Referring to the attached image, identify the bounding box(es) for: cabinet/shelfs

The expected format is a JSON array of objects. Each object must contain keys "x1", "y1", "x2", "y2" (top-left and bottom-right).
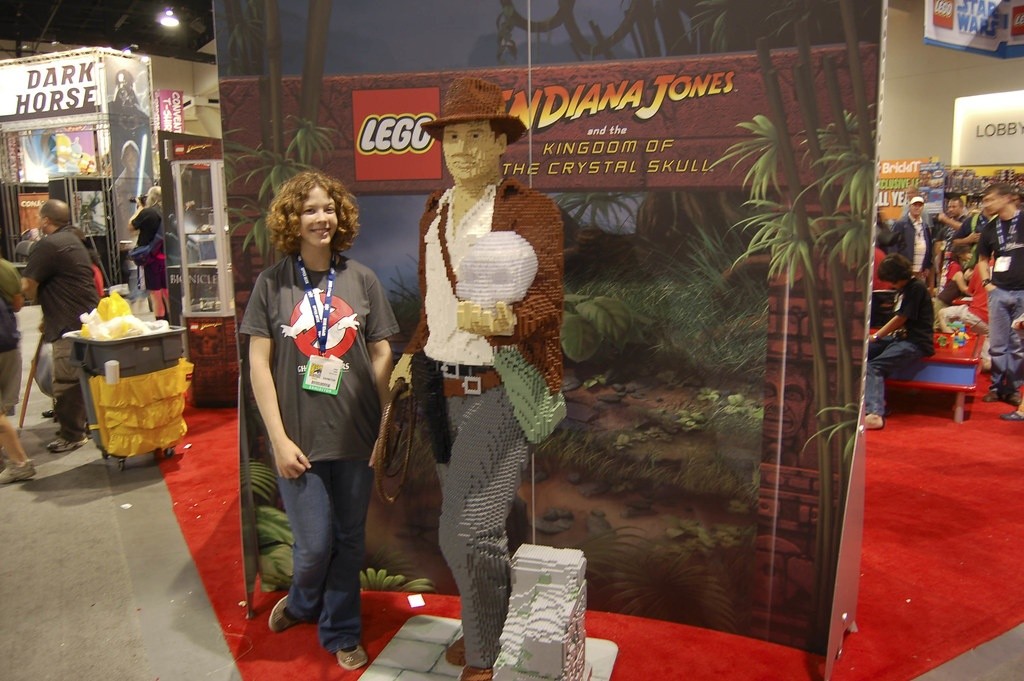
[{"x1": 172, "y1": 160, "x2": 236, "y2": 318}]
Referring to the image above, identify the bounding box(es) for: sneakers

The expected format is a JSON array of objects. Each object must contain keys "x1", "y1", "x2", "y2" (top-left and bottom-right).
[
  {"x1": 46, "y1": 435, "x2": 88, "y2": 452},
  {"x1": 0, "y1": 458, "x2": 37, "y2": 484},
  {"x1": 56, "y1": 421, "x2": 93, "y2": 439}
]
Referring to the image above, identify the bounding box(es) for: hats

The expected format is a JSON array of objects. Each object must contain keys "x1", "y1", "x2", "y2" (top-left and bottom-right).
[{"x1": 910, "y1": 196, "x2": 925, "y2": 205}]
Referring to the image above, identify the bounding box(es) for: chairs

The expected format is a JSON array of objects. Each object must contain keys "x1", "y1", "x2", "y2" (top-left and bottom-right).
[{"x1": 871, "y1": 289, "x2": 897, "y2": 327}]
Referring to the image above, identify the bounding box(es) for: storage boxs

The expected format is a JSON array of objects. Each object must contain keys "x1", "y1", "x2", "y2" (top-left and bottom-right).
[{"x1": 61, "y1": 326, "x2": 187, "y2": 378}]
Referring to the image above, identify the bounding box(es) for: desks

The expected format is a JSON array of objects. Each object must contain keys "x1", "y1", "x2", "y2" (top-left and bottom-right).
[{"x1": 870, "y1": 327, "x2": 985, "y2": 424}]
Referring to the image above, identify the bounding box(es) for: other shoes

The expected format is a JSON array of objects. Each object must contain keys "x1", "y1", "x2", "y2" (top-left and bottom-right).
[
  {"x1": 1005, "y1": 393, "x2": 1022, "y2": 405},
  {"x1": 268, "y1": 595, "x2": 301, "y2": 633},
  {"x1": 42, "y1": 409, "x2": 55, "y2": 417},
  {"x1": 337, "y1": 644, "x2": 368, "y2": 670},
  {"x1": 1000, "y1": 412, "x2": 1024, "y2": 420},
  {"x1": 983, "y1": 390, "x2": 1001, "y2": 402},
  {"x1": 864, "y1": 412, "x2": 883, "y2": 430}
]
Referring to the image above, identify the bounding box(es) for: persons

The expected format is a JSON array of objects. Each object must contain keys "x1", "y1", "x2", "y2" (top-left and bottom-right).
[
  {"x1": 114, "y1": 140, "x2": 151, "y2": 245},
  {"x1": 0, "y1": 199, "x2": 102, "y2": 483},
  {"x1": 388, "y1": 74, "x2": 568, "y2": 681},
  {"x1": 863, "y1": 182, "x2": 1024, "y2": 430},
  {"x1": 245, "y1": 173, "x2": 394, "y2": 671},
  {"x1": 127, "y1": 186, "x2": 169, "y2": 322},
  {"x1": 107, "y1": 70, "x2": 153, "y2": 174}
]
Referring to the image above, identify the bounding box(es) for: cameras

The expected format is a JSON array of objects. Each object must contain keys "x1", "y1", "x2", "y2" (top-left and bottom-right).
[{"x1": 130, "y1": 194, "x2": 148, "y2": 206}]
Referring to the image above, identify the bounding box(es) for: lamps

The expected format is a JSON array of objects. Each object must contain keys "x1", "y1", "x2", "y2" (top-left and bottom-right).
[
  {"x1": 122, "y1": 44, "x2": 139, "y2": 53},
  {"x1": 51, "y1": 41, "x2": 71, "y2": 51}
]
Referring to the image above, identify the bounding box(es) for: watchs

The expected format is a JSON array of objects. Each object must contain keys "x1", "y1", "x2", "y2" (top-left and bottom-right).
[
  {"x1": 872, "y1": 332, "x2": 881, "y2": 341},
  {"x1": 980, "y1": 279, "x2": 991, "y2": 287}
]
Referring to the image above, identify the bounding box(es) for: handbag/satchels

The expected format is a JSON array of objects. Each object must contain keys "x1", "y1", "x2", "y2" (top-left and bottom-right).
[
  {"x1": 937, "y1": 280, "x2": 961, "y2": 307},
  {"x1": 958, "y1": 214, "x2": 980, "y2": 269},
  {"x1": 127, "y1": 244, "x2": 154, "y2": 267}
]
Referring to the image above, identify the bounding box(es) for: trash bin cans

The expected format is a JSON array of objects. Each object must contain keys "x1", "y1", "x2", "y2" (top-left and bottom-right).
[{"x1": 62, "y1": 327, "x2": 188, "y2": 459}]
